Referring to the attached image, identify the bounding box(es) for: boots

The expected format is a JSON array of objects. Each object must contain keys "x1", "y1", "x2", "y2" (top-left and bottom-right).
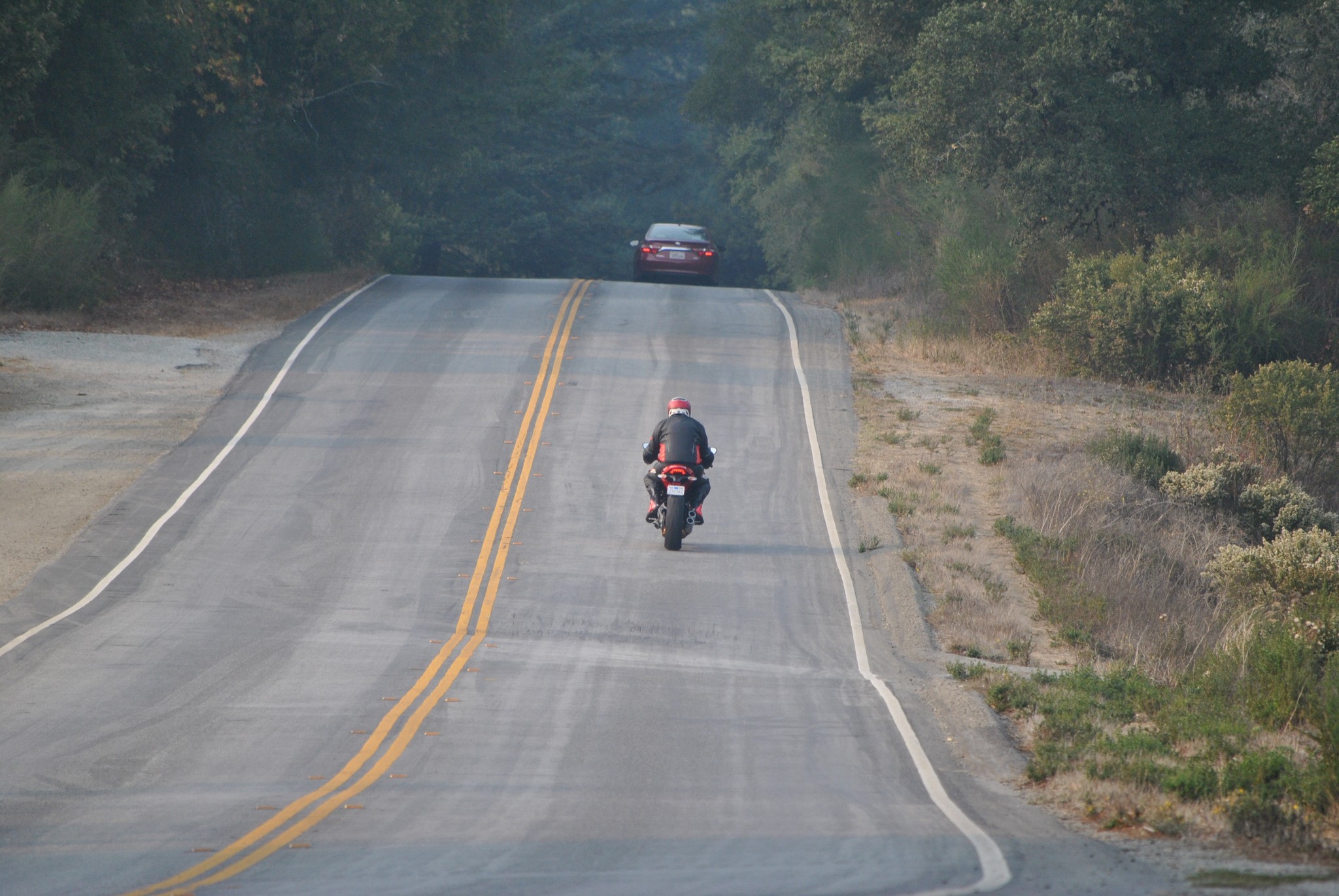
[
  {"x1": 646, "y1": 500, "x2": 657, "y2": 523},
  {"x1": 694, "y1": 504, "x2": 704, "y2": 525}
]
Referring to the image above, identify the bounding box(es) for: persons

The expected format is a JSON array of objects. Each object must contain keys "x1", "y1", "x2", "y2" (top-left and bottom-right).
[{"x1": 642, "y1": 396, "x2": 714, "y2": 524}]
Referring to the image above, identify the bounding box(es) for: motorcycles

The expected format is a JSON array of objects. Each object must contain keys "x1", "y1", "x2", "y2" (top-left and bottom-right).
[{"x1": 642, "y1": 441, "x2": 717, "y2": 551}]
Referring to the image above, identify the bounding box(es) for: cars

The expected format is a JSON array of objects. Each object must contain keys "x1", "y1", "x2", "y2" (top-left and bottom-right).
[{"x1": 630, "y1": 222, "x2": 726, "y2": 288}]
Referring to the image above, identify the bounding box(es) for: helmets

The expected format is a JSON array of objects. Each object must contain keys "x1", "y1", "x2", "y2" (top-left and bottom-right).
[{"x1": 668, "y1": 397, "x2": 692, "y2": 417}]
